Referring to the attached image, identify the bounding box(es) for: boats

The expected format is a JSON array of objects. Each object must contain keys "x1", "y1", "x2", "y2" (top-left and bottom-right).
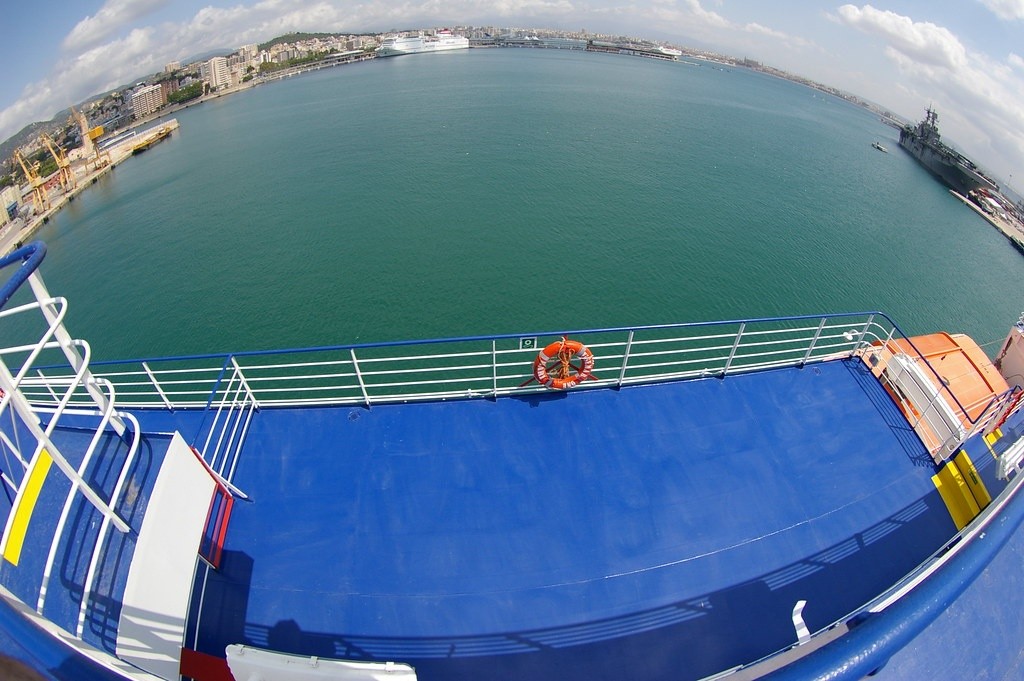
[
  {"x1": 133, "y1": 126, "x2": 171, "y2": 151},
  {"x1": 871, "y1": 141, "x2": 887, "y2": 153}
]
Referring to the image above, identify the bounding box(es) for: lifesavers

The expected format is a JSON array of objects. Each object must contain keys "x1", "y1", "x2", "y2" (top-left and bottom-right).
[{"x1": 533, "y1": 339, "x2": 594, "y2": 389}]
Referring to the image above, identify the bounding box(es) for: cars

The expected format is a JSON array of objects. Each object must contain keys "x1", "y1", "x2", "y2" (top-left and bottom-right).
[{"x1": 968, "y1": 185, "x2": 1024, "y2": 227}]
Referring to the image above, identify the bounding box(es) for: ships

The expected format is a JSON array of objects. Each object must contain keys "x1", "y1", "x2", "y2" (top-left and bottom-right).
[
  {"x1": 901, "y1": 104, "x2": 1000, "y2": 200},
  {"x1": 0, "y1": 236, "x2": 1024, "y2": 681},
  {"x1": 375, "y1": 31, "x2": 470, "y2": 57}
]
